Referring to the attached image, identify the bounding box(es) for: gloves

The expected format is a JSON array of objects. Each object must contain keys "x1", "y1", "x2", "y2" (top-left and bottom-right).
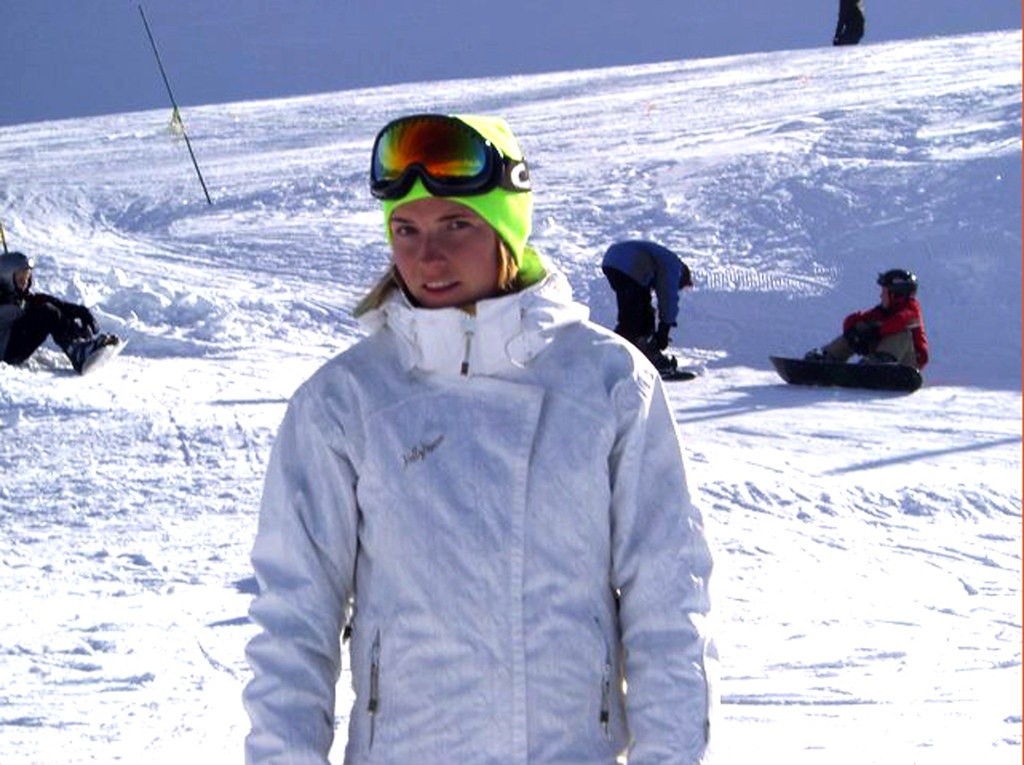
[
  {"x1": 855, "y1": 321, "x2": 881, "y2": 342},
  {"x1": 77, "y1": 305, "x2": 100, "y2": 334},
  {"x1": 655, "y1": 321, "x2": 671, "y2": 350},
  {"x1": 842, "y1": 325, "x2": 871, "y2": 356}
]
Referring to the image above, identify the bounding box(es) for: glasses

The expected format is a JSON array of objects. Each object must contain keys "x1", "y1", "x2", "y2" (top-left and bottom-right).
[{"x1": 368, "y1": 112, "x2": 533, "y2": 201}]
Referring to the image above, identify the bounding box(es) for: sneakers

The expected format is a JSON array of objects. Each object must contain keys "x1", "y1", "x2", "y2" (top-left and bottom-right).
[
  {"x1": 646, "y1": 339, "x2": 671, "y2": 372},
  {"x1": 66, "y1": 334, "x2": 107, "y2": 376},
  {"x1": 95, "y1": 329, "x2": 119, "y2": 374}
]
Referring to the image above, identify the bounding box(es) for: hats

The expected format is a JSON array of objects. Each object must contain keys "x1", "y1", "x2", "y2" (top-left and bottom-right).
[{"x1": 383, "y1": 116, "x2": 535, "y2": 269}]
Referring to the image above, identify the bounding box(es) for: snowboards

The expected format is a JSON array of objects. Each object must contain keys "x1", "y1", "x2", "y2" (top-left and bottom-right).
[
  {"x1": 659, "y1": 369, "x2": 695, "y2": 382},
  {"x1": 767, "y1": 356, "x2": 922, "y2": 394},
  {"x1": 80, "y1": 337, "x2": 132, "y2": 375}
]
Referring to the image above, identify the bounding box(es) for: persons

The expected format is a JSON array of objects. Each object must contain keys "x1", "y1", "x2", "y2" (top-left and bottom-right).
[
  {"x1": 600, "y1": 239, "x2": 693, "y2": 373},
  {"x1": 242, "y1": 110, "x2": 720, "y2": 765},
  {"x1": 832, "y1": 0, "x2": 866, "y2": 46},
  {"x1": 0, "y1": 251, "x2": 125, "y2": 377},
  {"x1": 803, "y1": 268, "x2": 930, "y2": 369}
]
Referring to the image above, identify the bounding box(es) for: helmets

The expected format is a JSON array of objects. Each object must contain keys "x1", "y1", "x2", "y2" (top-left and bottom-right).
[
  {"x1": 876, "y1": 268, "x2": 918, "y2": 298},
  {"x1": 0, "y1": 251, "x2": 34, "y2": 295}
]
